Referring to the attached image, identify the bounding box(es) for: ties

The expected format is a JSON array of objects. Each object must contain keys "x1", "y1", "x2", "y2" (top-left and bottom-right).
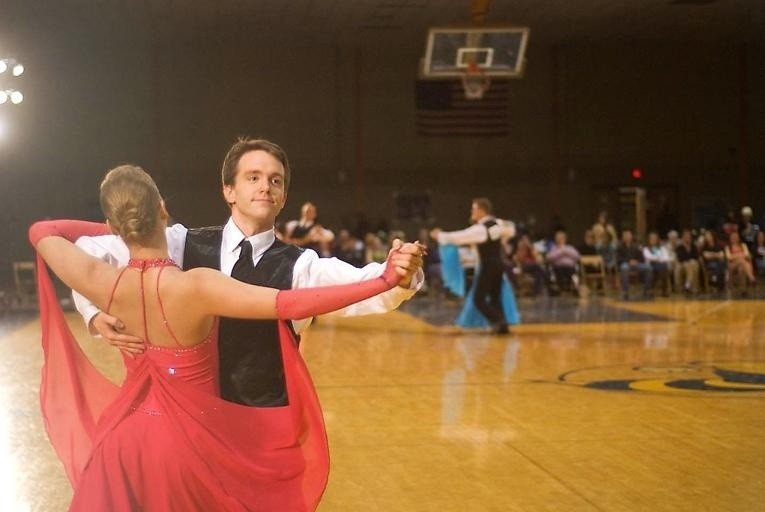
[{"x1": 231, "y1": 241, "x2": 254, "y2": 283}]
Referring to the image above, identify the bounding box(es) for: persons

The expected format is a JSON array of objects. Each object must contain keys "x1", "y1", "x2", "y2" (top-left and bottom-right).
[
  {"x1": 71, "y1": 136, "x2": 426, "y2": 408},
  {"x1": 284, "y1": 199, "x2": 759, "y2": 332},
  {"x1": 31, "y1": 165, "x2": 423, "y2": 512}
]
